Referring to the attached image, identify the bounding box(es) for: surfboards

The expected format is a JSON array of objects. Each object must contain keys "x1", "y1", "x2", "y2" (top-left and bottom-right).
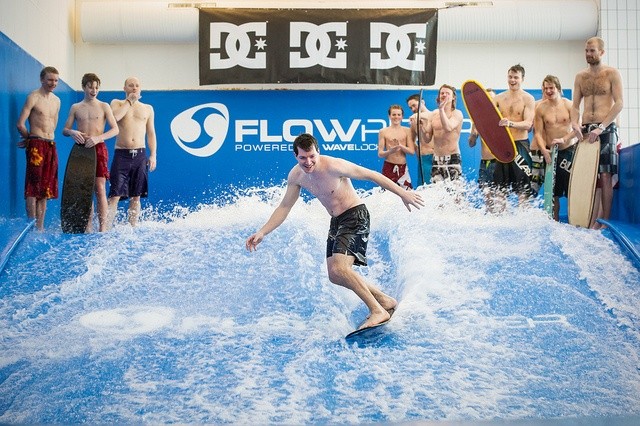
[
  {"x1": 544, "y1": 144, "x2": 559, "y2": 221},
  {"x1": 344, "y1": 306, "x2": 396, "y2": 339},
  {"x1": 460, "y1": 79, "x2": 518, "y2": 164},
  {"x1": 567, "y1": 132, "x2": 601, "y2": 230},
  {"x1": 416, "y1": 88, "x2": 425, "y2": 186},
  {"x1": 60, "y1": 142, "x2": 97, "y2": 235}
]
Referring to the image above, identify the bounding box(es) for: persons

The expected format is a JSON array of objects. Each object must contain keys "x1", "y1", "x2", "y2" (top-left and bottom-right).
[
  {"x1": 572, "y1": 35, "x2": 623, "y2": 231},
  {"x1": 246, "y1": 132, "x2": 426, "y2": 331},
  {"x1": 16, "y1": 65, "x2": 61, "y2": 231},
  {"x1": 108, "y1": 76, "x2": 157, "y2": 230},
  {"x1": 488, "y1": 64, "x2": 535, "y2": 211},
  {"x1": 418, "y1": 83, "x2": 463, "y2": 179},
  {"x1": 528, "y1": 82, "x2": 552, "y2": 201},
  {"x1": 468, "y1": 88, "x2": 503, "y2": 184},
  {"x1": 377, "y1": 103, "x2": 415, "y2": 192},
  {"x1": 405, "y1": 94, "x2": 435, "y2": 182},
  {"x1": 62, "y1": 71, "x2": 121, "y2": 231},
  {"x1": 535, "y1": 74, "x2": 582, "y2": 223}
]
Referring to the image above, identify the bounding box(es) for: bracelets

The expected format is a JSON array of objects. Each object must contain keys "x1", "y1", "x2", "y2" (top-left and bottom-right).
[{"x1": 509, "y1": 121, "x2": 514, "y2": 127}]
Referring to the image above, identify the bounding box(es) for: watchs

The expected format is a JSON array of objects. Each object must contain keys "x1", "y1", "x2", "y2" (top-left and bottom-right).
[{"x1": 598, "y1": 124, "x2": 606, "y2": 131}]
